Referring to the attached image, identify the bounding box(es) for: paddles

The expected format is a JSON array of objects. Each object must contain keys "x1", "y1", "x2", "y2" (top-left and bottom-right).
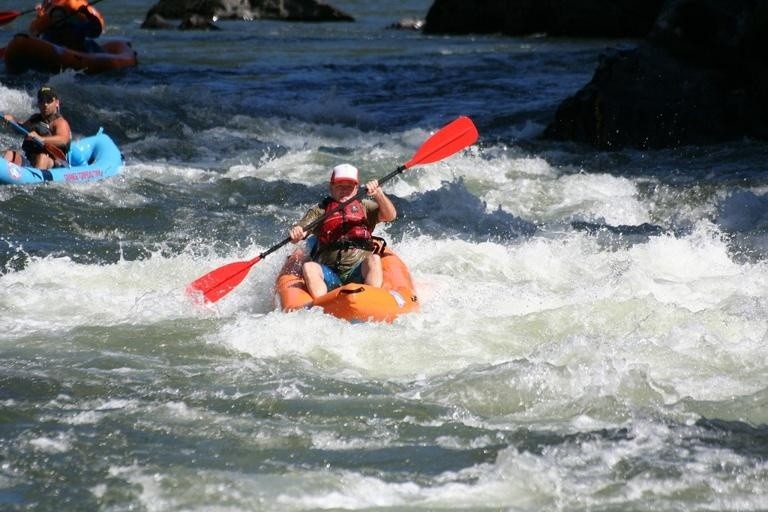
[
  {"x1": 0, "y1": 113, "x2": 66, "y2": 161},
  {"x1": 1, "y1": 9, "x2": 35, "y2": 26},
  {"x1": 188, "y1": 115, "x2": 479, "y2": 305}
]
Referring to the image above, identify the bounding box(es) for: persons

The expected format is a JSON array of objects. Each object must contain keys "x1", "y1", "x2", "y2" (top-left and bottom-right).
[
  {"x1": 288, "y1": 164, "x2": 397, "y2": 301},
  {"x1": 3, "y1": 87, "x2": 72, "y2": 170},
  {"x1": 30, "y1": 0, "x2": 103, "y2": 54}
]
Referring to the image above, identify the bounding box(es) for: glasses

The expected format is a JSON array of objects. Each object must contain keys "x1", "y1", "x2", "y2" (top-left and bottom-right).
[{"x1": 41, "y1": 99, "x2": 54, "y2": 104}]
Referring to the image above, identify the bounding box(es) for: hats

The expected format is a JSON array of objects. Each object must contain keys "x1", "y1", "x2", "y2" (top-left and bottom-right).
[
  {"x1": 38, "y1": 87, "x2": 57, "y2": 102},
  {"x1": 331, "y1": 164, "x2": 361, "y2": 185}
]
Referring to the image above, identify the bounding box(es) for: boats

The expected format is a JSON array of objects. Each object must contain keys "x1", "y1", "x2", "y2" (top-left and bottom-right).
[
  {"x1": 274, "y1": 234, "x2": 419, "y2": 327},
  {"x1": 1, "y1": 33, "x2": 138, "y2": 78},
  {"x1": 0, "y1": 129, "x2": 122, "y2": 186}
]
